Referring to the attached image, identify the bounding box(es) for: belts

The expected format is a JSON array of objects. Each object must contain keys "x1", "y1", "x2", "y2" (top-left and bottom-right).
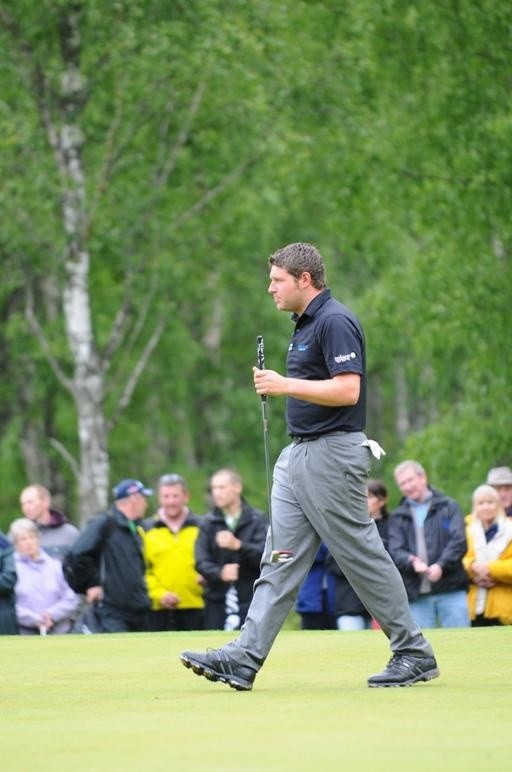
[{"x1": 292, "y1": 430, "x2": 336, "y2": 444}]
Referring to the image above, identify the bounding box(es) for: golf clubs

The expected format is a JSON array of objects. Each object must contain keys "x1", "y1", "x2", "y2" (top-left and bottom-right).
[{"x1": 254, "y1": 334, "x2": 295, "y2": 565}]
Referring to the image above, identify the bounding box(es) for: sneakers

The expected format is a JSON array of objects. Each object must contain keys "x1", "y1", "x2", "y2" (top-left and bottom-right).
[
  {"x1": 179, "y1": 647, "x2": 256, "y2": 691},
  {"x1": 366, "y1": 654, "x2": 440, "y2": 687}
]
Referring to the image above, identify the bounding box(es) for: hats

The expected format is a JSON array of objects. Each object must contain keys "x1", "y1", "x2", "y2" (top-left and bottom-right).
[
  {"x1": 486, "y1": 466, "x2": 512, "y2": 486},
  {"x1": 112, "y1": 478, "x2": 155, "y2": 500}
]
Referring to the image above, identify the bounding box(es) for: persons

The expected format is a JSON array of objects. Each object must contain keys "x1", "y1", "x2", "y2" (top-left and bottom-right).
[
  {"x1": 294, "y1": 460, "x2": 512, "y2": 631},
  {"x1": 0, "y1": 469, "x2": 268, "y2": 635},
  {"x1": 179, "y1": 242, "x2": 442, "y2": 693}
]
